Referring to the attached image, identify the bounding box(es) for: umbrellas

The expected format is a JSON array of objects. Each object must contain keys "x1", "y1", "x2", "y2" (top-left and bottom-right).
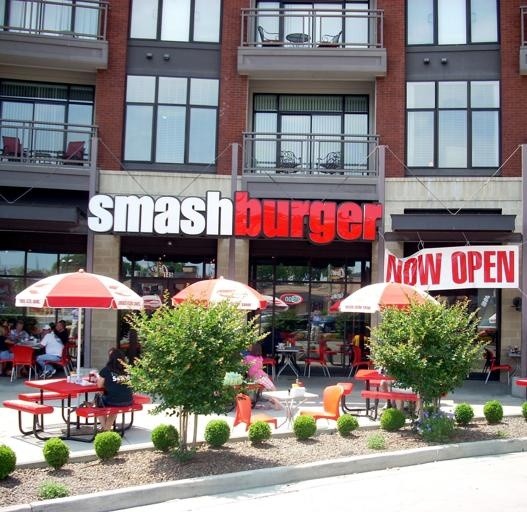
[
  {"x1": 167, "y1": 275, "x2": 268, "y2": 314},
  {"x1": 14, "y1": 268, "x2": 148, "y2": 436},
  {"x1": 262, "y1": 293, "x2": 291, "y2": 313},
  {"x1": 141, "y1": 292, "x2": 162, "y2": 308},
  {"x1": 327, "y1": 281, "x2": 446, "y2": 408}
]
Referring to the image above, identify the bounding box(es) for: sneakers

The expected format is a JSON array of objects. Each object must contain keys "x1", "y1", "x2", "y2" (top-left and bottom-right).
[{"x1": 46, "y1": 368, "x2": 56, "y2": 378}]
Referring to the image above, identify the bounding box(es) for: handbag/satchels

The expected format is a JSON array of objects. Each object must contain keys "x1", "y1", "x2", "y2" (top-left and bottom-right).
[{"x1": 94, "y1": 393, "x2": 104, "y2": 408}]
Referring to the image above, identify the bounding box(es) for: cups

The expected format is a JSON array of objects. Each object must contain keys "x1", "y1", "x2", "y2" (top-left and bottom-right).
[{"x1": 67, "y1": 376, "x2": 71, "y2": 383}]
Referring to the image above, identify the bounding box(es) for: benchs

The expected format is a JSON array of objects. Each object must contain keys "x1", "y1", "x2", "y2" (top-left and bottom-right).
[
  {"x1": 337, "y1": 382, "x2": 423, "y2": 419},
  {"x1": 2, "y1": 391, "x2": 152, "y2": 445}
]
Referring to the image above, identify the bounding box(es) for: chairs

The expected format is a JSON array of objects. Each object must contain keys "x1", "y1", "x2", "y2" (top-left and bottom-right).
[
  {"x1": 233, "y1": 392, "x2": 278, "y2": 433},
  {"x1": 0, "y1": 340, "x2": 80, "y2": 382},
  {"x1": 55, "y1": 140, "x2": 84, "y2": 166},
  {"x1": 481, "y1": 346, "x2": 512, "y2": 385},
  {"x1": 300, "y1": 382, "x2": 345, "y2": 425},
  {"x1": 313, "y1": 30, "x2": 344, "y2": 48},
  {"x1": 258, "y1": 339, "x2": 373, "y2": 382},
  {"x1": 0, "y1": 134, "x2": 28, "y2": 161},
  {"x1": 314, "y1": 152, "x2": 342, "y2": 176},
  {"x1": 276, "y1": 150, "x2": 302, "y2": 173},
  {"x1": 256, "y1": 26, "x2": 284, "y2": 49}
]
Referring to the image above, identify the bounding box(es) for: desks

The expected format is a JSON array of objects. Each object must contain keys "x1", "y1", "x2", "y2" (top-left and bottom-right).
[
  {"x1": 286, "y1": 33, "x2": 310, "y2": 48},
  {"x1": 24, "y1": 376, "x2": 107, "y2": 437},
  {"x1": 260, "y1": 387, "x2": 319, "y2": 430},
  {"x1": 352, "y1": 368, "x2": 402, "y2": 419}
]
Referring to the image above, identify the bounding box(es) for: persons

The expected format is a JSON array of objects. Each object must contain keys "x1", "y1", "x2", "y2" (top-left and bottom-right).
[
  {"x1": 82, "y1": 347, "x2": 114, "y2": 409},
  {"x1": 377, "y1": 361, "x2": 420, "y2": 412},
  {"x1": 241, "y1": 343, "x2": 283, "y2": 410},
  {"x1": 91, "y1": 347, "x2": 132, "y2": 432},
  {"x1": 0, "y1": 319, "x2": 68, "y2": 379},
  {"x1": 261, "y1": 328, "x2": 371, "y2": 373},
  {"x1": 114, "y1": 325, "x2": 144, "y2": 362}
]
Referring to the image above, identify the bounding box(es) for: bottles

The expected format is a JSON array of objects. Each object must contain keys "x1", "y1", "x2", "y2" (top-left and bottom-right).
[
  {"x1": 89, "y1": 372, "x2": 95, "y2": 384},
  {"x1": 287, "y1": 381, "x2": 305, "y2": 397}
]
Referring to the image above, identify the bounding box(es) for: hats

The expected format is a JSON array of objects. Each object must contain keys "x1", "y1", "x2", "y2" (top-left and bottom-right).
[{"x1": 41, "y1": 324, "x2": 52, "y2": 330}]
